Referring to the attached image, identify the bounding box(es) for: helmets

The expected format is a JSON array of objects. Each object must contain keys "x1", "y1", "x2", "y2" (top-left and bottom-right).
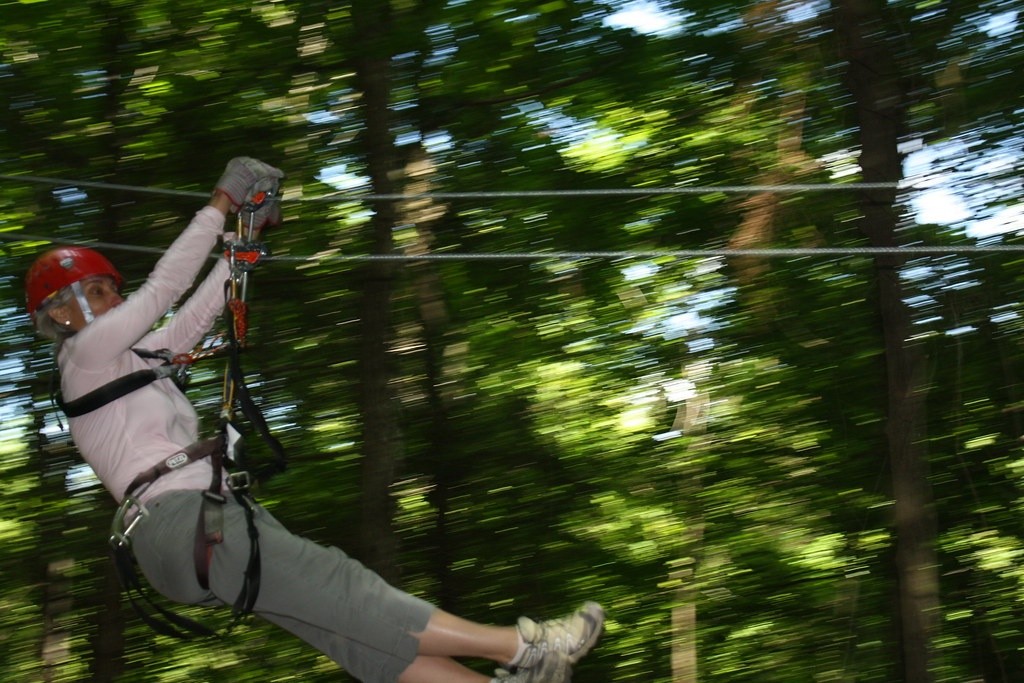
[{"x1": 25, "y1": 246, "x2": 122, "y2": 323}]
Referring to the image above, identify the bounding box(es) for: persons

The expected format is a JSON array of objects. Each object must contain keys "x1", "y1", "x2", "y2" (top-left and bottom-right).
[{"x1": 25, "y1": 156, "x2": 604, "y2": 683}]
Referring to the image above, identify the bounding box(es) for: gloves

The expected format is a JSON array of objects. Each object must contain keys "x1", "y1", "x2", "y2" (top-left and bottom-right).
[
  {"x1": 214, "y1": 156, "x2": 283, "y2": 213},
  {"x1": 240, "y1": 175, "x2": 280, "y2": 229}
]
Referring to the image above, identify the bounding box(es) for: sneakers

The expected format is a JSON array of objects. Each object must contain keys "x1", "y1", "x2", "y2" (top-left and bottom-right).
[
  {"x1": 494, "y1": 652, "x2": 572, "y2": 683},
  {"x1": 511, "y1": 602, "x2": 603, "y2": 668}
]
